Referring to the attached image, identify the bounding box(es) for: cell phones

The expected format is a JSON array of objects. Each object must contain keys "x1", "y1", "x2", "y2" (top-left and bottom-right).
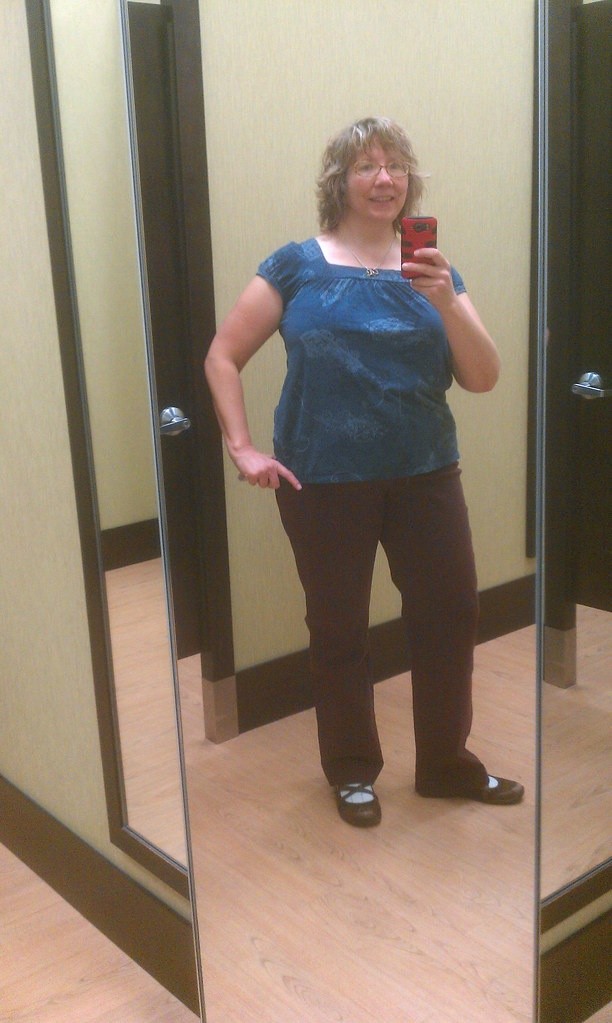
[{"x1": 400, "y1": 216, "x2": 439, "y2": 280}]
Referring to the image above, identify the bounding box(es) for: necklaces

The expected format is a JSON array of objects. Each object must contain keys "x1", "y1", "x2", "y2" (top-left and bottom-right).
[{"x1": 335, "y1": 230, "x2": 398, "y2": 278}]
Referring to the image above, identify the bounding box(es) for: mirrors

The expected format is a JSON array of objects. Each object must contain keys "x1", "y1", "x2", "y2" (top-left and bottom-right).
[
  {"x1": 534, "y1": 0, "x2": 612, "y2": 939},
  {"x1": 118, "y1": 0, "x2": 545, "y2": 1023},
  {"x1": 27, "y1": 1, "x2": 196, "y2": 897}
]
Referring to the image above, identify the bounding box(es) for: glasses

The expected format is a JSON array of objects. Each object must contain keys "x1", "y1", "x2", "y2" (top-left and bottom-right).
[{"x1": 348, "y1": 157, "x2": 412, "y2": 179}]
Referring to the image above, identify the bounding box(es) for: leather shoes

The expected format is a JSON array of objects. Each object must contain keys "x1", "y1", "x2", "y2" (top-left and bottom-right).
[
  {"x1": 334, "y1": 781, "x2": 382, "y2": 829},
  {"x1": 463, "y1": 773, "x2": 525, "y2": 805}
]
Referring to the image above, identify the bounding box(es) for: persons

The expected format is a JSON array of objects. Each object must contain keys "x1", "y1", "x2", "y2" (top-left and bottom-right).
[{"x1": 202, "y1": 116, "x2": 528, "y2": 827}]
